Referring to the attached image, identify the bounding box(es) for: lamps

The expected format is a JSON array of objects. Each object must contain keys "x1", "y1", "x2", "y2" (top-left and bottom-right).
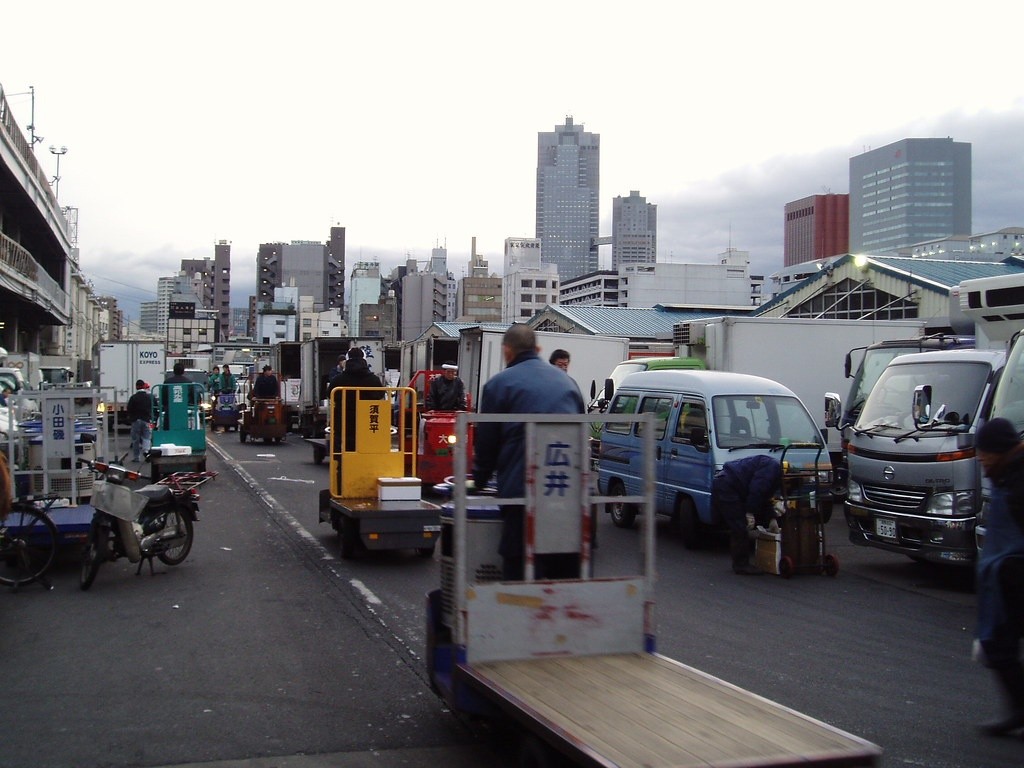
[{"x1": 816, "y1": 261, "x2": 833, "y2": 277}]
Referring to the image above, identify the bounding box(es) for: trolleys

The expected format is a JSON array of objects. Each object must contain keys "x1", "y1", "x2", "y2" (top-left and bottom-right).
[{"x1": 774, "y1": 443, "x2": 836, "y2": 576}]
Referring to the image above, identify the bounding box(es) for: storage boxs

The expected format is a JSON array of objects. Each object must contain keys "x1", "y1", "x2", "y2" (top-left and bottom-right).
[
  {"x1": 151, "y1": 446, "x2": 192, "y2": 455},
  {"x1": 378, "y1": 477, "x2": 422, "y2": 501}
]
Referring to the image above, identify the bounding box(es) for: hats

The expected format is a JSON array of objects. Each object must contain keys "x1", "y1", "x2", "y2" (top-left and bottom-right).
[
  {"x1": 145, "y1": 383, "x2": 150, "y2": 388},
  {"x1": 136, "y1": 379, "x2": 145, "y2": 388}
]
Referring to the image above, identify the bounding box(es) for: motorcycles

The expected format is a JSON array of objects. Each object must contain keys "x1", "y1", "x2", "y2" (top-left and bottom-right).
[{"x1": 78, "y1": 449, "x2": 219, "y2": 591}]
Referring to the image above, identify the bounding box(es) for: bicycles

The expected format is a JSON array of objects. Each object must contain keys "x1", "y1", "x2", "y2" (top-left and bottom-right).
[{"x1": 0, "y1": 493, "x2": 65, "y2": 591}]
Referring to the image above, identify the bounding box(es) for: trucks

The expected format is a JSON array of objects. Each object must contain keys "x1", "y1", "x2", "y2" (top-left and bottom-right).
[
  {"x1": 455, "y1": 272, "x2": 1024, "y2": 590},
  {"x1": 38, "y1": 336, "x2": 402, "y2": 442}
]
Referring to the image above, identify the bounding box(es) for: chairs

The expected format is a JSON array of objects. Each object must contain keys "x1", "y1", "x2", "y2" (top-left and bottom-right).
[
  {"x1": 683, "y1": 411, "x2": 707, "y2": 433},
  {"x1": 730, "y1": 416, "x2": 752, "y2": 438}
]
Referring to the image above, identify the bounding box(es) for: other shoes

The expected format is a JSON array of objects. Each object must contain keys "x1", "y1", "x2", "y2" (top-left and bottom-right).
[
  {"x1": 143, "y1": 452, "x2": 150, "y2": 463},
  {"x1": 738, "y1": 565, "x2": 763, "y2": 576},
  {"x1": 131, "y1": 459, "x2": 139, "y2": 462},
  {"x1": 978, "y1": 717, "x2": 1024, "y2": 741}
]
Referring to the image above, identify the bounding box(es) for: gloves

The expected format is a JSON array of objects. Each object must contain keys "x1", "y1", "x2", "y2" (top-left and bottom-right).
[
  {"x1": 769, "y1": 515, "x2": 781, "y2": 535},
  {"x1": 746, "y1": 512, "x2": 754, "y2": 530}
]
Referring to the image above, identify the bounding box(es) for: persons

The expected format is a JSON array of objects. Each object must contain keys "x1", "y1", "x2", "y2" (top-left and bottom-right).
[
  {"x1": 972, "y1": 417, "x2": 1024, "y2": 731},
  {"x1": 328, "y1": 355, "x2": 347, "y2": 382},
  {"x1": 709, "y1": 455, "x2": 801, "y2": 575},
  {"x1": 425, "y1": 361, "x2": 468, "y2": 412},
  {"x1": 252, "y1": 365, "x2": 281, "y2": 402},
  {"x1": 470, "y1": 323, "x2": 599, "y2": 581},
  {"x1": 549, "y1": 349, "x2": 570, "y2": 373},
  {"x1": 162, "y1": 362, "x2": 202, "y2": 430},
  {"x1": 219, "y1": 364, "x2": 237, "y2": 394},
  {"x1": 2, "y1": 387, "x2": 13, "y2": 398},
  {"x1": 126, "y1": 379, "x2": 159, "y2": 461},
  {"x1": 207, "y1": 366, "x2": 224, "y2": 396},
  {"x1": 327, "y1": 348, "x2": 385, "y2": 495}
]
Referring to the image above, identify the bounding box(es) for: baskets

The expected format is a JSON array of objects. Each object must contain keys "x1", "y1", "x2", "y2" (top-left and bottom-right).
[{"x1": 90, "y1": 481, "x2": 149, "y2": 521}]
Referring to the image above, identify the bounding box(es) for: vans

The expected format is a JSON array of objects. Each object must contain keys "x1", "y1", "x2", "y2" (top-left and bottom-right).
[{"x1": 598, "y1": 369, "x2": 835, "y2": 548}]
